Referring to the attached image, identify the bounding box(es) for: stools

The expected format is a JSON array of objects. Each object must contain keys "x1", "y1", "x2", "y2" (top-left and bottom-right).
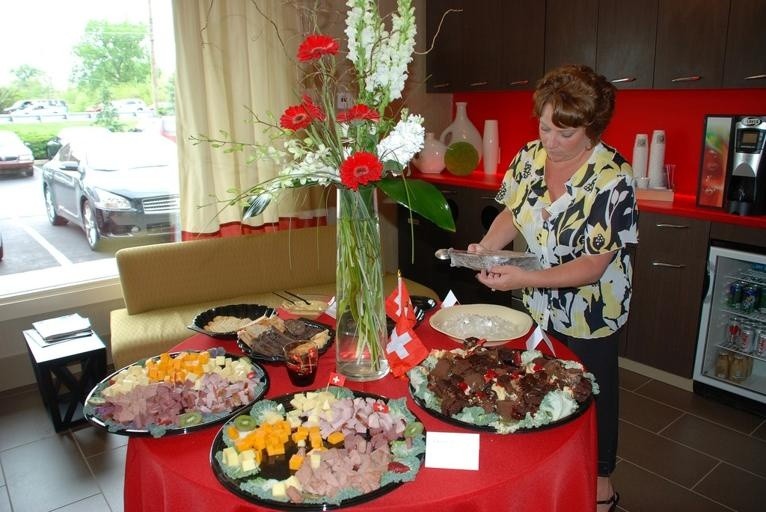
[{"x1": 21, "y1": 329, "x2": 107, "y2": 434}]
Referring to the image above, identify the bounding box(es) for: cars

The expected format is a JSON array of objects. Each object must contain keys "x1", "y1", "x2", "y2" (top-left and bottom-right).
[
  {"x1": 115, "y1": 99, "x2": 147, "y2": 111},
  {"x1": 42, "y1": 132, "x2": 179, "y2": 249},
  {"x1": 46, "y1": 126, "x2": 111, "y2": 160},
  {"x1": 0, "y1": 130, "x2": 34, "y2": 176},
  {"x1": 10, "y1": 105, "x2": 69, "y2": 119}
]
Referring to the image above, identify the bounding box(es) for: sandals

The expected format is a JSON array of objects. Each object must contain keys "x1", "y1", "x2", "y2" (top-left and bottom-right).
[{"x1": 597, "y1": 491, "x2": 620, "y2": 512}]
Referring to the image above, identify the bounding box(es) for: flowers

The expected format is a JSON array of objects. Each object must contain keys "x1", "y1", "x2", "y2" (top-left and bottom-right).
[{"x1": 186, "y1": 0, "x2": 463, "y2": 370}]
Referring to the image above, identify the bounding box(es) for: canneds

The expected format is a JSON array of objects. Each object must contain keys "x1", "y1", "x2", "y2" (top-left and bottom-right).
[{"x1": 715, "y1": 350, "x2": 753, "y2": 381}]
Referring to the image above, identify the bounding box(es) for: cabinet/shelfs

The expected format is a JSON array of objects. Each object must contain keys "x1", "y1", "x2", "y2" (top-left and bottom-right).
[
  {"x1": 544, "y1": 0, "x2": 656, "y2": 91},
  {"x1": 653, "y1": 0, "x2": 766, "y2": 90},
  {"x1": 426, "y1": 1, "x2": 545, "y2": 94},
  {"x1": 396, "y1": 180, "x2": 720, "y2": 395}
]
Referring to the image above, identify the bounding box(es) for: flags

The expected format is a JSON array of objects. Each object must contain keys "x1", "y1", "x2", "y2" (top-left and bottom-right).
[
  {"x1": 385, "y1": 270, "x2": 417, "y2": 329},
  {"x1": 384, "y1": 302, "x2": 430, "y2": 378}
]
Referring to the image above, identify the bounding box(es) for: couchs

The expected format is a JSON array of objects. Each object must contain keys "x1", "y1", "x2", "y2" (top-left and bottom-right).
[{"x1": 109, "y1": 223, "x2": 441, "y2": 372}]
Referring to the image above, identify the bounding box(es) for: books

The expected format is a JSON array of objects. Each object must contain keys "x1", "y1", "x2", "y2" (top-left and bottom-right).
[{"x1": 32, "y1": 313, "x2": 92, "y2": 339}]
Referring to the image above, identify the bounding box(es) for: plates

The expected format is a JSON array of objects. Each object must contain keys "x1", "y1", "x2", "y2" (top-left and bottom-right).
[
  {"x1": 409, "y1": 349, "x2": 599, "y2": 434},
  {"x1": 187, "y1": 304, "x2": 276, "y2": 340},
  {"x1": 235, "y1": 318, "x2": 337, "y2": 363},
  {"x1": 385, "y1": 295, "x2": 435, "y2": 335},
  {"x1": 209, "y1": 388, "x2": 427, "y2": 511},
  {"x1": 429, "y1": 305, "x2": 533, "y2": 347},
  {"x1": 84, "y1": 350, "x2": 269, "y2": 436}
]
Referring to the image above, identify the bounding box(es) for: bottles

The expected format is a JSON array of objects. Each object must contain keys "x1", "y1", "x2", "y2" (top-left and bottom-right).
[
  {"x1": 728, "y1": 275, "x2": 766, "y2": 314},
  {"x1": 727, "y1": 316, "x2": 766, "y2": 357}
]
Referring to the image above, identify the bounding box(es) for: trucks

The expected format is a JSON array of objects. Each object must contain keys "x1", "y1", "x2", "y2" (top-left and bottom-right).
[{"x1": 3, "y1": 99, "x2": 69, "y2": 114}]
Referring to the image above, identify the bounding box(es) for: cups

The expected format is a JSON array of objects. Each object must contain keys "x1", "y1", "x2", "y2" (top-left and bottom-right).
[
  {"x1": 632, "y1": 134, "x2": 647, "y2": 177},
  {"x1": 634, "y1": 176, "x2": 651, "y2": 189},
  {"x1": 283, "y1": 340, "x2": 317, "y2": 387},
  {"x1": 648, "y1": 131, "x2": 664, "y2": 188}
]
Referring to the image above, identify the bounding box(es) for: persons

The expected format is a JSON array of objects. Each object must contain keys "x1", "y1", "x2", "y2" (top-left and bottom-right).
[{"x1": 467, "y1": 65, "x2": 639, "y2": 512}]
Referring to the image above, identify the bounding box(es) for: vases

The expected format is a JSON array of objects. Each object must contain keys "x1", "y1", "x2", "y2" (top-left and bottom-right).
[{"x1": 335, "y1": 188, "x2": 391, "y2": 382}]
